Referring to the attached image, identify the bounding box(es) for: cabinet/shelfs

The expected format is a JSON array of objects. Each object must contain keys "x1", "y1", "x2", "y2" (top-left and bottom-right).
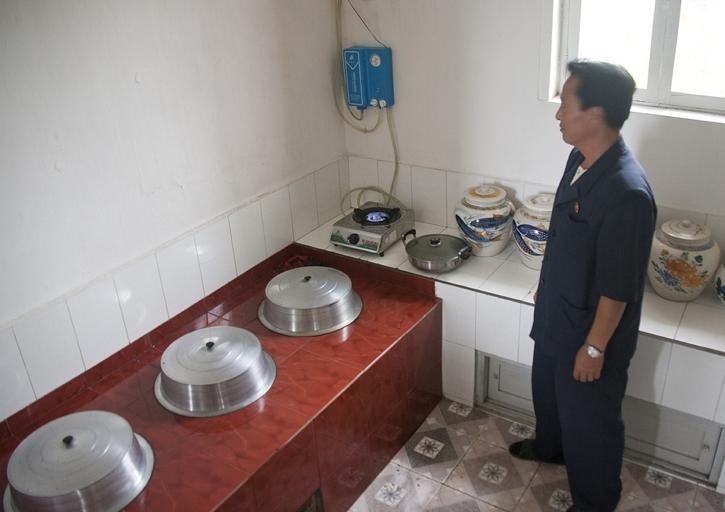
[{"x1": 488, "y1": 355, "x2": 722, "y2": 474}]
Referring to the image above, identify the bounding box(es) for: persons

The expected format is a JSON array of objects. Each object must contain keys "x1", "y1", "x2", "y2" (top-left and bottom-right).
[{"x1": 508, "y1": 57, "x2": 657, "y2": 511}]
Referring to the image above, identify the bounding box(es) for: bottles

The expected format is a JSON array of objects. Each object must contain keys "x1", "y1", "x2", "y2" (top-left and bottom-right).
[{"x1": 453, "y1": 184, "x2": 725, "y2": 301}]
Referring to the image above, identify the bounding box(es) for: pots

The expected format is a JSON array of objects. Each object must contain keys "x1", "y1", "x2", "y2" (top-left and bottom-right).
[{"x1": 400, "y1": 229, "x2": 473, "y2": 274}]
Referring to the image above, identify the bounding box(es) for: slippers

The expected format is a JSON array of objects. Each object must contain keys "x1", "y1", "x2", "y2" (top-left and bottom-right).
[{"x1": 508, "y1": 439, "x2": 568, "y2": 464}]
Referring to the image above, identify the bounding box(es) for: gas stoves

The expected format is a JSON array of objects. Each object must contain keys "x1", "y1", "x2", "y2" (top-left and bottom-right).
[{"x1": 330, "y1": 201, "x2": 415, "y2": 257}]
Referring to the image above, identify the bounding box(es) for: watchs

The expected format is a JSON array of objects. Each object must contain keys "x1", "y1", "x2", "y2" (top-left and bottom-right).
[{"x1": 581, "y1": 341, "x2": 609, "y2": 360}]
